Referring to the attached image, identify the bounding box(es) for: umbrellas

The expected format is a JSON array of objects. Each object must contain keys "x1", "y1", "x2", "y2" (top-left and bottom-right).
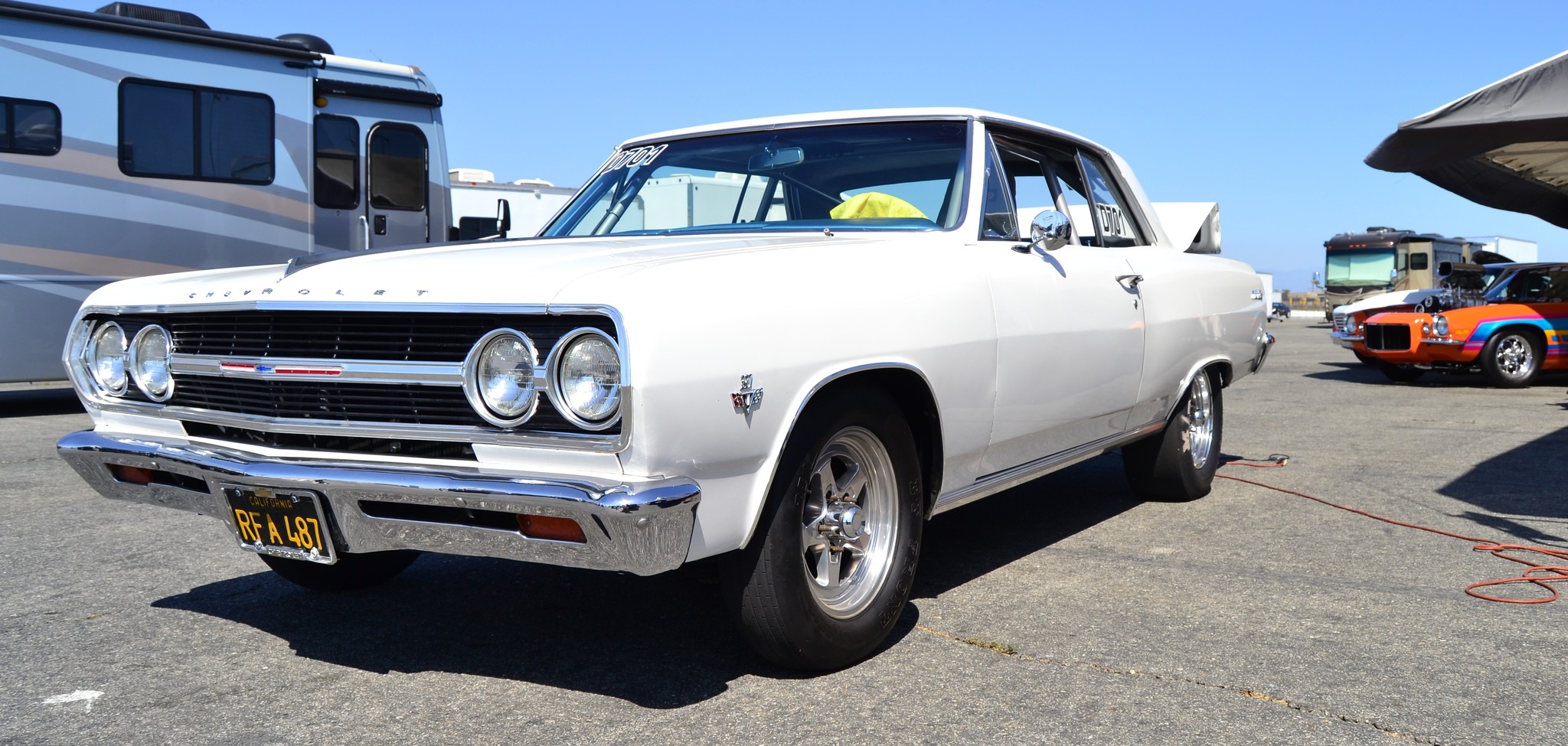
[{"x1": 1365, "y1": 45, "x2": 1568, "y2": 230}]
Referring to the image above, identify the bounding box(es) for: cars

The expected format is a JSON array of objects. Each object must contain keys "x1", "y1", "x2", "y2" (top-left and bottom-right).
[
  {"x1": 1271, "y1": 302, "x2": 1291, "y2": 318},
  {"x1": 45, "y1": 102, "x2": 1278, "y2": 677},
  {"x1": 1330, "y1": 261, "x2": 1568, "y2": 390}
]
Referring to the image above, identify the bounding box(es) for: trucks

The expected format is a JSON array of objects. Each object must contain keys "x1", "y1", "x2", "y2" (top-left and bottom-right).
[{"x1": 1313, "y1": 225, "x2": 1487, "y2": 328}]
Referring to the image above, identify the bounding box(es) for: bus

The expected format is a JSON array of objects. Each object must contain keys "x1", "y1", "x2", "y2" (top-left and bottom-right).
[{"x1": 1, "y1": 2, "x2": 511, "y2": 401}]
